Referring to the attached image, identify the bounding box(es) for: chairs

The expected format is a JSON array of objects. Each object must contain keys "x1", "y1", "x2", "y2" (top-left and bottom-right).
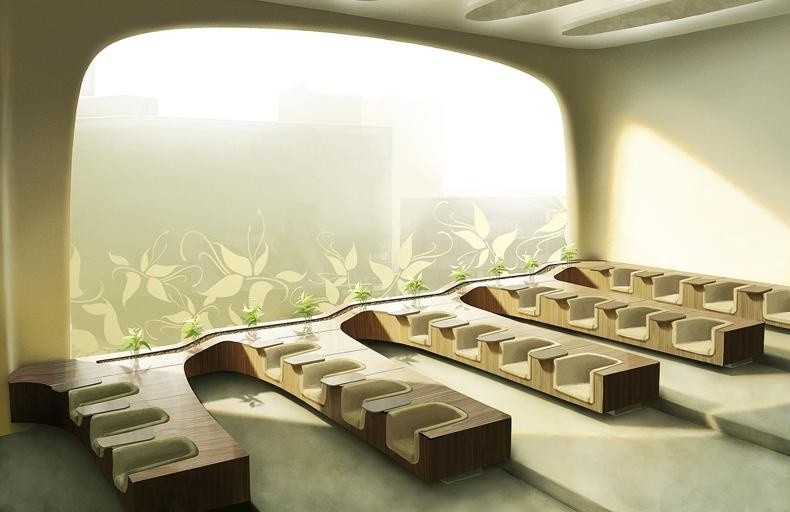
[
  {"x1": 464, "y1": 273, "x2": 769, "y2": 372},
  {"x1": 10, "y1": 347, "x2": 263, "y2": 511},
  {"x1": 363, "y1": 294, "x2": 664, "y2": 418},
  {"x1": 567, "y1": 258, "x2": 790, "y2": 333},
  {"x1": 229, "y1": 313, "x2": 516, "y2": 494}
]
